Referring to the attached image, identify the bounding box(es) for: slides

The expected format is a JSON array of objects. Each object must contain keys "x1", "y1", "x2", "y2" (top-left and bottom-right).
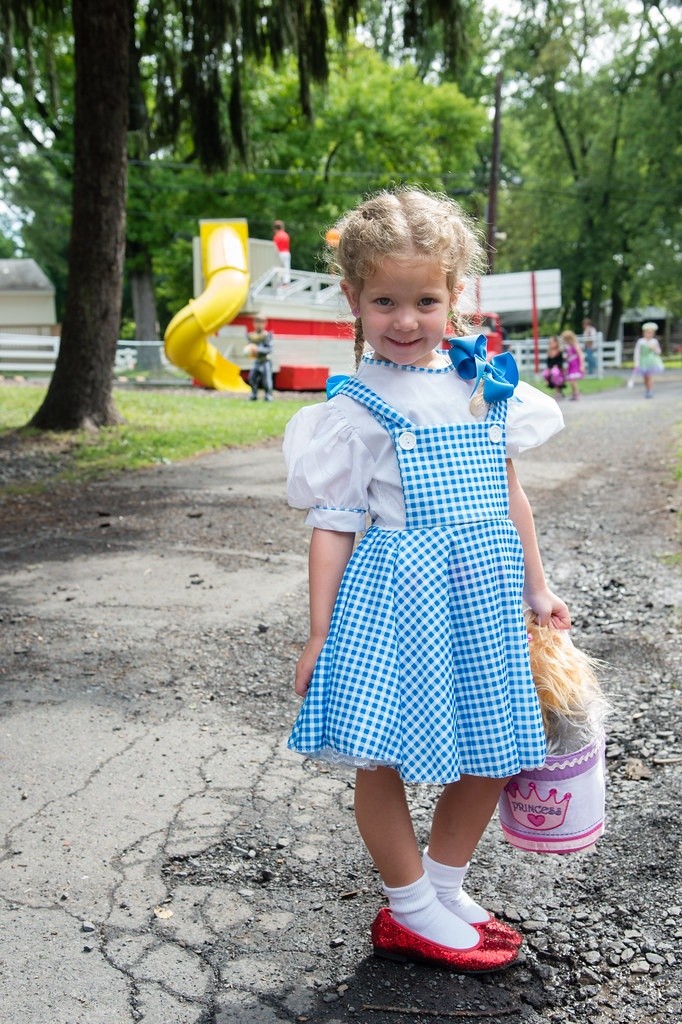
[{"x1": 162, "y1": 228, "x2": 254, "y2": 395}]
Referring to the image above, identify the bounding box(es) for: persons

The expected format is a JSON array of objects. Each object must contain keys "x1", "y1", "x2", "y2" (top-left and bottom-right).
[
  {"x1": 632, "y1": 322, "x2": 664, "y2": 399},
  {"x1": 271, "y1": 220, "x2": 291, "y2": 287},
  {"x1": 545, "y1": 335, "x2": 566, "y2": 398},
  {"x1": 245, "y1": 315, "x2": 273, "y2": 401},
  {"x1": 561, "y1": 330, "x2": 586, "y2": 401},
  {"x1": 582, "y1": 319, "x2": 597, "y2": 375},
  {"x1": 282, "y1": 187, "x2": 570, "y2": 976}
]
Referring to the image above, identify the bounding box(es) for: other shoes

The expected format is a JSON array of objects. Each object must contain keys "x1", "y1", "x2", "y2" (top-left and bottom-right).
[
  {"x1": 372, "y1": 905, "x2": 518, "y2": 973},
  {"x1": 251, "y1": 395, "x2": 258, "y2": 401},
  {"x1": 469, "y1": 911, "x2": 523, "y2": 948},
  {"x1": 265, "y1": 394, "x2": 273, "y2": 402}
]
